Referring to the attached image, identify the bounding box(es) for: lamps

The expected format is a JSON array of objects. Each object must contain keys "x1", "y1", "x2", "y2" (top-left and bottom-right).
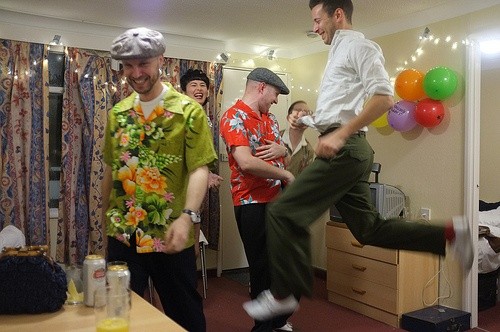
[
  {"x1": 421, "y1": 27, "x2": 430, "y2": 38},
  {"x1": 52, "y1": 34, "x2": 61, "y2": 44},
  {"x1": 267, "y1": 50, "x2": 274, "y2": 61},
  {"x1": 216, "y1": 53, "x2": 228, "y2": 65}
]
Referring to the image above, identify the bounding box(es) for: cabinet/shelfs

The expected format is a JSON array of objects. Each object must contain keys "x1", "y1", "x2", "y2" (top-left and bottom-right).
[{"x1": 325, "y1": 220, "x2": 442, "y2": 328}]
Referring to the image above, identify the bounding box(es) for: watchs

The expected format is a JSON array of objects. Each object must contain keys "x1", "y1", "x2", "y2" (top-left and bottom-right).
[{"x1": 184, "y1": 209, "x2": 201, "y2": 224}]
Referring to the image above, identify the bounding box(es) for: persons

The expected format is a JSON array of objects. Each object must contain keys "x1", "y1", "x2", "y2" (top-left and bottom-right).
[
  {"x1": 219, "y1": 67, "x2": 295, "y2": 332},
  {"x1": 241, "y1": 0, "x2": 475, "y2": 320},
  {"x1": 279, "y1": 100, "x2": 314, "y2": 178},
  {"x1": 101, "y1": 28, "x2": 219, "y2": 332},
  {"x1": 180, "y1": 69, "x2": 224, "y2": 188}
]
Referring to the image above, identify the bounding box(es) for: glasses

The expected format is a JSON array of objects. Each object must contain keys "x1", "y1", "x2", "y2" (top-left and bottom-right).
[{"x1": 297, "y1": 110, "x2": 313, "y2": 115}]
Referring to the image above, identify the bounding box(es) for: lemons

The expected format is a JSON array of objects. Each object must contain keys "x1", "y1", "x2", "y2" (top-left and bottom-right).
[{"x1": 66, "y1": 276, "x2": 85, "y2": 301}]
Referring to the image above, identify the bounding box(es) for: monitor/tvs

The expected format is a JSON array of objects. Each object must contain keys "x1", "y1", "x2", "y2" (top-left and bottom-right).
[{"x1": 330, "y1": 182, "x2": 406, "y2": 222}]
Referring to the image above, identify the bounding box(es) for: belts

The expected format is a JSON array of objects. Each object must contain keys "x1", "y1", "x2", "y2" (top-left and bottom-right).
[{"x1": 321, "y1": 128, "x2": 359, "y2": 136}]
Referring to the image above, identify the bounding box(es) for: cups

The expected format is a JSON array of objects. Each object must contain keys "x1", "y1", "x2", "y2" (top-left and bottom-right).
[
  {"x1": 65, "y1": 265, "x2": 84, "y2": 305},
  {"x1": 93, "y1": 286, "x2": 131, "y2": 332}
]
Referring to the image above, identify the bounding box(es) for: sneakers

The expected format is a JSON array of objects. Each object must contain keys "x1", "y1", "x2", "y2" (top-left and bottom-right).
[
  {"x1": 243, "y1": 289, "x2": 298, "y2": 319},
  {"x1": 450, "y1": 215, "x2": 474, "y2": 273}
]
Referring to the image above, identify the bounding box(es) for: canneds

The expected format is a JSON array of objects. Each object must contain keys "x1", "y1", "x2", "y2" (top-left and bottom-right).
[{"x1": 83, "y1": 255, "x2": 106, "y2": 307}]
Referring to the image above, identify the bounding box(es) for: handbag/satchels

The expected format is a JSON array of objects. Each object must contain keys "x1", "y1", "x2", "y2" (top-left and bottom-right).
[{"x1": 0, "y1": 245, "x2": 68, "y2": 315}]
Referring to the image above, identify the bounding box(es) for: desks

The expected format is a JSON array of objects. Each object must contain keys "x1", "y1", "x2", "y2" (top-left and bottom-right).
[{"x1": 0, "y1": 291, "x2": 188, "y2": 332}]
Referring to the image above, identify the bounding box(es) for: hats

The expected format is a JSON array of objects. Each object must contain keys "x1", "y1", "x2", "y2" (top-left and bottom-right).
[
  {"x1": 247, "y1": 68, "x2": 290, "y2": 95},
  {"x1": 180, "y1": 69, "x2": 210, "y2": 92},
  {"x1": 111, "y1": 28, "x2": 166, "y2": 59}
]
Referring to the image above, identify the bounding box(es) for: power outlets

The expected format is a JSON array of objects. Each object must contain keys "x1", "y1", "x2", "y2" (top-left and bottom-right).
[{"x1": 418, "y1": 207, "x2": 431, "y2": 222}]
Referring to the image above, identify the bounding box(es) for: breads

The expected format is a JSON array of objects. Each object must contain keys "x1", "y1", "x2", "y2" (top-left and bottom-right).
[{"x1": 105, "y1": 264, "x2": 131, "y2": 318}]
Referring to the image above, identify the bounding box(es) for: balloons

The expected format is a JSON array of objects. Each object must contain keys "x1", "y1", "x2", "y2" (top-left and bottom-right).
[
  {"x1": 414, "y1": 98, "x2": 445, "y2": 128},
  {"x1": 387, "y1": 100, "x2": 418, "y2": 133},
  {"x1": 425, "y1": 66, "x2": 458, "y2": 100},
  {"x1": 364, "y1": 95, "x2": 395, "y2": 128},
  {"x1": 395, "y1": 67, "x2": 425, "y2": 102}
]
